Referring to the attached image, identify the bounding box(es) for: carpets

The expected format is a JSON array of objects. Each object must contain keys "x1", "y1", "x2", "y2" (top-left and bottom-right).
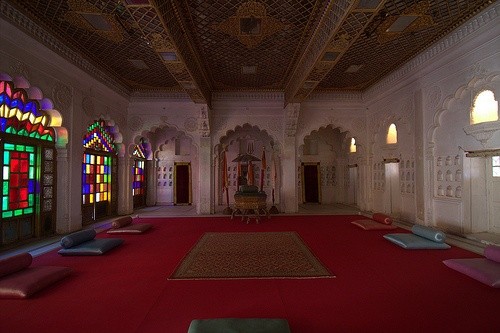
[{"x1": 166, "y1": 231, "x2": 337, "y2": 280}]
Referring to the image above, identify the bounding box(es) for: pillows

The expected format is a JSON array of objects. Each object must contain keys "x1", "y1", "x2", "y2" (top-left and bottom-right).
[
  {"x1": 0, "y1": 252, "x2": 33, "y2": 279},
  {"x1": 59, "y1": 229, "x2": 96, "y2": 249},
  {"x1": 371, "y1": 212, "x2": 392, "y2": 225},
  {"x1": 112, "y1": 216, "x2": 133, "y2": 229},
  {"x1": 484, "y1": 243, "x2": 500, "y2": 262},
  {"x1": 411, "y1": 223, "x2": 446, "y2": 244}
]
